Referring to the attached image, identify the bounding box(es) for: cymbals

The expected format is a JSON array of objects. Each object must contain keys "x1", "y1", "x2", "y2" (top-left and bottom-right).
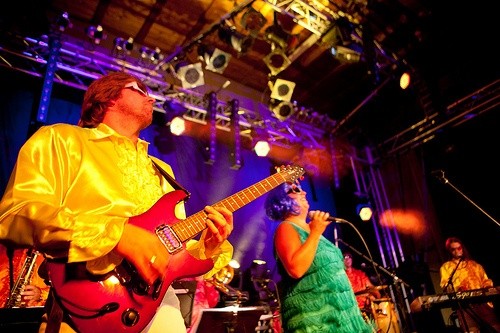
[{"x1": 354, "y1": 284, "x2": 388, "y2": 296}]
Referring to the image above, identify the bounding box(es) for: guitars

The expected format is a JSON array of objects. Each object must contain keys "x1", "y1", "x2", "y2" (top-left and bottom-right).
[{"x1": 47, "y1": 162, "x2": 307, "y2": 333}]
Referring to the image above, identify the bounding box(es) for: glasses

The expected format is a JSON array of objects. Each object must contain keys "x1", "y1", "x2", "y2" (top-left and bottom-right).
[
  {"x1": 287, "y1": 184, "x2": 300, "y2": 194},
  {"x1": 125, "y1": 81, "x2": 147, "y2": 96}
]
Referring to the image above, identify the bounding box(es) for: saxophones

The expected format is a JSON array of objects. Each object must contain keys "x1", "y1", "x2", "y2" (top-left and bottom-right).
[{"x1": 2, "y1": 248, "x2": 41, "y2": 308}]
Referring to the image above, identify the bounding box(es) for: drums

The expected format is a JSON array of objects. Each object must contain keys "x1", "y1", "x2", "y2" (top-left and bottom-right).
[{"x1": 368, "y1": 298, "x2": 401, "y2": 333}]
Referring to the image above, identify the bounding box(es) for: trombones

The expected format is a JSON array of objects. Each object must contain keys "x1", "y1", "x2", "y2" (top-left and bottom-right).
[{"x1": 204, "y1": 264, "x2": 243, "y2": 303}]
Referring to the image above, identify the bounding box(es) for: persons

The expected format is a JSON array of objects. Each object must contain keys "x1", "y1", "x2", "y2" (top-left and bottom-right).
[
  {"x1": 440, "y1": 237, "x2": 500, "y2": 333},
  {"x1": 264, "y1": 182, "x2": 374, "y2": 333},
  {"x1": 0, "y1": 242, "x2": 51, "y2": 308},
  {"x1": 0, "y1": 71, "x2": 233, "y2": 333},
  {"x1": 342, "y1": 249, "x2": 381, "y2": 309}
]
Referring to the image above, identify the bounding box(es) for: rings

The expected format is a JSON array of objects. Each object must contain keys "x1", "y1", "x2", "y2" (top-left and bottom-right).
[{"x1": 151, "y1": 255, "x2": 157, "y2": 264}]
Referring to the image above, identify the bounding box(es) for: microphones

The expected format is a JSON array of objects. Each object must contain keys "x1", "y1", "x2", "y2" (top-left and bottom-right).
[
  {"x1": 251, "y1": 276, "x2": 274, "y2": 283},
  {"x1": 309, "y1": 211, "x2": 347, "y2": 224}
]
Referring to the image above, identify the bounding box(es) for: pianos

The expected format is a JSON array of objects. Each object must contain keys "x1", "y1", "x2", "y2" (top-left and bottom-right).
[{"x1": 408, "y1": 285, "x2": 500, "y2": 333}]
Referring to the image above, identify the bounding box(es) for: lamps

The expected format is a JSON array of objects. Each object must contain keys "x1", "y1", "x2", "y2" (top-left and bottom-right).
[
  {"x1": 139, "y1": 46, "x2": 165, "y2": 65},
  {"x1": 250, "y1": 127, "x2": 271, "y2": 157},
  {"x1": 222, "y1": 258, "x2": 240, "y2": 284},
  {"x1": 55, "y1": 11, "x2": 74, "y2": 32},
  {"x1": 167, "y1": 0, "x2": 412, "y2": 123},
  {"x1": 114, "y1": 36, "x2": 136, "y2": 56},
  {"x1": 85, "y1": 25, "x2": 107, "y2": 45},
  {"x1": 250, "y1": 260, "x2": 267, "y2": 282},
  {"x1": 355, "y1": 202, "x2": 373, "y2": 222},
  {"x1": 164, "y1": 104, "x2": 186, "y2": 136}
]
